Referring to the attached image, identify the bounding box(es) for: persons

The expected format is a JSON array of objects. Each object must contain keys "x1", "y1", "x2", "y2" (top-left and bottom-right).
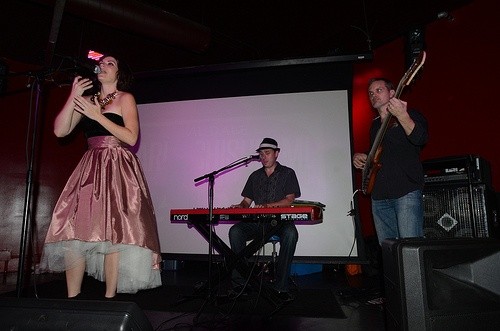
[
  {"x1": 226, "y1": 137, "x2": 302, "y2": 300},
  {"x1": 38, "y1": 52, "x2": 164, "y2": 302},
  {"x1": 351, "y1": 77, "x2": 434, "y2": 305}
]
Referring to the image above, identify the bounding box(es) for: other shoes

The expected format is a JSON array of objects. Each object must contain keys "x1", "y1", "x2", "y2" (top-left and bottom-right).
[
  {"x1": 270, "y1": 287, "x2": 289, "y2": 302},
  {"x1": 228, "y1": 279, "x2": 248, "y2": 296}
]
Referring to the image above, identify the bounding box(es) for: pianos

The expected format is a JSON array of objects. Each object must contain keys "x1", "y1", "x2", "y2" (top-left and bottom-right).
[{"x1": 170, "y1": 205, "x2": 323, "y2": 317}]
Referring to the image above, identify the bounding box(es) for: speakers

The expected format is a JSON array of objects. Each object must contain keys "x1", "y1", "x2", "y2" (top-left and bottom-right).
[
  {"x1": 420, "y1": 180, "x2": 500, "y2": 238},
  {"x1": 381, "y1": 235, "x2": 500, "y2": 331},
  {"x1": 0, "y1": 296, "x2": 154, "y2": 331}
]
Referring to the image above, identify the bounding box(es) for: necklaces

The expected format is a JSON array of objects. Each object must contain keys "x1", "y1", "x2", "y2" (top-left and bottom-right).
[{"x1": 91, "y1": 90, "x2": 118, "y2": 112}]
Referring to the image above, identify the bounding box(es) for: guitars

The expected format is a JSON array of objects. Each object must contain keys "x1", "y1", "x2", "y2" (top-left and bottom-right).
[{"x1": 362, "y1": 50, "x2": 427, "y2": 196}]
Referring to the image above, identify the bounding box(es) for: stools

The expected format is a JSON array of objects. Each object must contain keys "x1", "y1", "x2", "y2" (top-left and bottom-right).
[{"x1": 261, "y1": 235, "x2": 279, "y2": 280}]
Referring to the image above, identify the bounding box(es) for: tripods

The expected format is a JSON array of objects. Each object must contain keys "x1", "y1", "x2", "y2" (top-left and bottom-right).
[{"x1": 182, "y1": 159, "x2": 253, "y2": 325}]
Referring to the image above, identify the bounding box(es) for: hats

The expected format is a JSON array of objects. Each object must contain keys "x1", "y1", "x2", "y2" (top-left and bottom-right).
[{"x1": 256, "y1": 138, "x2": 281, "y2": 153}]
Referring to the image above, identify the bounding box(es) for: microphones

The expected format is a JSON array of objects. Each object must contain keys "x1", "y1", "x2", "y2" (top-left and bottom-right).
[
  {"x1": 251, "y1": 155, "x2": 261, "y2": 160},
  {"x1": 67, "y1": 56, "x2": 101, "y2": 73}
]
